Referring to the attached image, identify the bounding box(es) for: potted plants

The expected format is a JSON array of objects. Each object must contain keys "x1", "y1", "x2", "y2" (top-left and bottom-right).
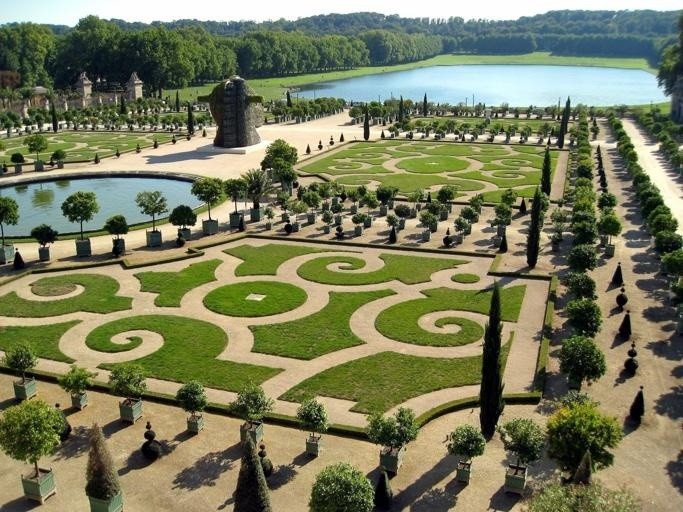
[
  {"x1": 174, "y1": 379, "x2": 207, "y2": 435},
  {"x1": 1, "y1": 168, "x2": 278, "y2": 271},
  {"x1": 5, "y1": 340, "x2": 37, "y2": 400},
  {"x1": 1, "y1": 400, "x2": 66, "y2": 507},
  {"x1": 229, "y1": 380, "x2": 275, "y2": 449},
  {"x1": 264, "y1": 87, "x2": 628, "y2": 273},
  {"x1": 76, "y1": 421, "x2": 125, "y2": 512},
  {"x1": 106, "y1": 360, "x2": 146, "y2": 425},
  {"x1": 441, "y1": 423, "x2": 485, "y2": 486},
  {"x1": 495, "y1": 416, "x2": 545, "y2": 497},
  {"x1": 0, "y1": 89, "x2": 208, "y2": 175},
  {"x1": 294, "y1": 397, "x2": 328, "y2": 459},
  {"x1": 518, "y1": 272, "x2": 628, "y2": 511},
  {"x1": 56, "y1": 363, "x2": 100, "y2": 412},
  {"x1": 363, "y1": 400, "x2": 419, "y2": 476}
]
